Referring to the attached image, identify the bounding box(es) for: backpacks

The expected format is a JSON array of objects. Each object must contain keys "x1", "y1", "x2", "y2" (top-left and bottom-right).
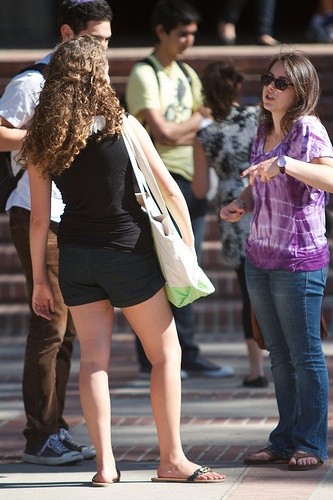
[{"x1": 1, "y1": 63, "x2": 49, "y2": 211}]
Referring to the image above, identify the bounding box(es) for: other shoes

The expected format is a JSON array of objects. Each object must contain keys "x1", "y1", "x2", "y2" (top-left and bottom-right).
[
  {"x1": 139, "y1": 365, "x2": 234, "y2": 380},
  {"x1": 242, "y1": 374, "x2": 269, "y2": 388}
]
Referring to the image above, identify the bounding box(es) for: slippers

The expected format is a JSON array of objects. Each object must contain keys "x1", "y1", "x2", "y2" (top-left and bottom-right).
[
  {"x1": 288, "y1": 451, "x2": 324, "y2": 471},
  {"x1": 92, "y1": 469, "x2": 121, "y2": 487},
  {"x1": 244, "y1": 447, "x2": 291, "y2": 464},
  {"x1": 150, "y1": 467, "x2": 227, "y2": 483}
]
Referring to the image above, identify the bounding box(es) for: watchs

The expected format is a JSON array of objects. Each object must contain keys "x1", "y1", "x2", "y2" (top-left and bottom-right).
[{"x1": 276, "y1": 154, "x2": 287, "y2": 174}]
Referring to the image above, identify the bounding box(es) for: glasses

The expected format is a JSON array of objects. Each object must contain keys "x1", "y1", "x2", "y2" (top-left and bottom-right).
[{"x1": 259, "y1": 72, "x2": 295, "y2": 90}]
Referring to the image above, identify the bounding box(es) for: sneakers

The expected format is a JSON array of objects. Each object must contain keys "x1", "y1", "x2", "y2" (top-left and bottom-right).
[{"x1": 23, "y1": 428, "x2": 97, "y2": 465}]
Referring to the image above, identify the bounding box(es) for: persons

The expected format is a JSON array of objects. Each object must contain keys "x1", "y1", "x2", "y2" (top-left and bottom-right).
[
  {"x1": 220, "y1": 51, "x2": 332, "y2": 471},
  {"x1": 124, "y1": 10, "x2": 235, "y2": 383},
  {"x1": 182, "y1": 56, "x2": 271, "y2": 386},
  {"x1": 16, "y1": 36, "x2": 228, "y2": 486},
  {"x1": 306, "y1": 0, "x2": 333, "y2": 43},
  {"x1": 218, "y1": 0, "x2": 281, "y2": 46},
  {"x1": 0, "y1": 0, "x2": 117, "y2": 466}
]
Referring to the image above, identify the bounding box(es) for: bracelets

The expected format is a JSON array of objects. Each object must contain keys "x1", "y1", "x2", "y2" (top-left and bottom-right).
[{"x1": 232, "y1": 198, "x2": 248, "y2": 212}]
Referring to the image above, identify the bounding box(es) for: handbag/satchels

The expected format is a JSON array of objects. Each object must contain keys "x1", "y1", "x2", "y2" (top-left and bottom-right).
[{"x1": 119, "y1": 116, "x2": 216, "y2": 308}]
